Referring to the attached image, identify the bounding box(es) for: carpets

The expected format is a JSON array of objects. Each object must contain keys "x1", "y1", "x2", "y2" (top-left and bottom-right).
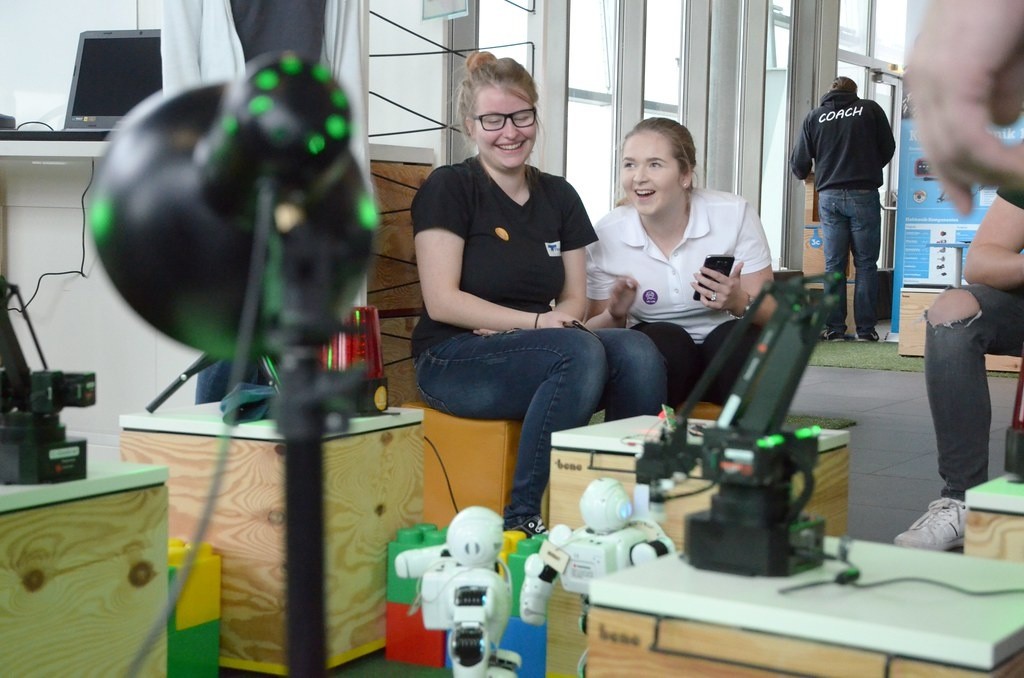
[
  {"x1": 783, "y1": 413, "x2": 856, "y2": 430},
  {"x1": 807, "y1": 339, "x2": 1022, "y2": 379}
]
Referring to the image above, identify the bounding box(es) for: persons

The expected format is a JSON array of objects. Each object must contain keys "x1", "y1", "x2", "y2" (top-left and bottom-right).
[
  {"x1": 396, "y1": 506, "x2": 522, "y2": 678},
  {"x1": 159, "y1": 0, "x2": 372, "y2": 405},
  {"x1": 586, "y1": 118, "x2": 777, "y2": 410},
  {"x1": 789, "y1": 77, "x2": 895, "y2": 343},
  {"x1": 519, "y1": 477, "x2": 673, "y2": 677},
  {"x1": 412, "y1": 51, "x2": 668, "y2": 536},
  {"x1": 893, "y1": 185, "x2": 1023, "y2": 550},
  {"x1": 905, "y1": 0, "x2": 1023, "y2": 214}
]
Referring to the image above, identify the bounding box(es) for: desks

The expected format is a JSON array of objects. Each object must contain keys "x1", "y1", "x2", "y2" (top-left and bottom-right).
[
  {"x1": 899, "y1": 285, "x2": 951, "y2": 356},
  {"x1": 964, "y1": 472, "x2": 1024, "y2": 563},
  {"x1": 551, "y1": 415, "x2": 850, "y2": 557},
  {"x1": 586, "y1": 536, "x2": 1024, "y2": 678},
  {"x1": 0, "y1": 463, "x2": 170, "y2": 678},
  {"x1": 118, "y1": 400, "x2": 424, "y2": 676}
]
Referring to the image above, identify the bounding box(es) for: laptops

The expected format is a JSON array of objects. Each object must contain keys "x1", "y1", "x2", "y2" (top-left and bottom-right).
[{"x1": 1, "y1": 30, "x2": 163, "y2": 141}]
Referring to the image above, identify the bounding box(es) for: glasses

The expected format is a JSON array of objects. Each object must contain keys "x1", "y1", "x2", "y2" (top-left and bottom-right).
[{"x1": 474, "y1": 106, "x2": 537, "y2": 131}]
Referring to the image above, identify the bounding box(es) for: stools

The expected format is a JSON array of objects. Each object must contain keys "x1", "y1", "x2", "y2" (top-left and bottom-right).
[{"x1": 402, "y1": 399, "x2": 525, "y2": 531}]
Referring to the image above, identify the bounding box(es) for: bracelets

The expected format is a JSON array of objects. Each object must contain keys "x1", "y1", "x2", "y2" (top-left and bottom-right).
[
  {"x1": 535, "y1": 313, "x2": 539, "y2": 329},
  {"x1": 726, "y1": 296, "x2": 753, "y2": 319}
]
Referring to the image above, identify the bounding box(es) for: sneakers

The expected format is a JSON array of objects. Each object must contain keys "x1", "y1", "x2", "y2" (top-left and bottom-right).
[
  {"x1": 512, "y1": 514, "x2": 548, "y2": 538},
  {"x1": 893, "y1": 496, "x2": 967, "y2": 550},
  {"x1": 856, "y1": 329, "x2": 879, "y2": 343},
  {"x1": 819, "y1": 329, "x2": 845, "y2": 342}
]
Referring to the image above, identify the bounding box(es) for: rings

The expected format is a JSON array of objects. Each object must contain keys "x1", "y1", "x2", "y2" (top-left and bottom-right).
[{"x1": 711, "y1": 292, "x2": 716, "y2": 301}]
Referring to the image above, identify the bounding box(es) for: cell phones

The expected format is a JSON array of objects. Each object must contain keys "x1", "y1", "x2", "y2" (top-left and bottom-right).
[{"x1": 693, "y1": 255, "x2": 734, "y2": 301}]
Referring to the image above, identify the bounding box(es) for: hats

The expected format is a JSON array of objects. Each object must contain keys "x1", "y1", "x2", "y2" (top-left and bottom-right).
[{"x1": 832, "y1": 77, "x2": 857, "y2": 92}]
